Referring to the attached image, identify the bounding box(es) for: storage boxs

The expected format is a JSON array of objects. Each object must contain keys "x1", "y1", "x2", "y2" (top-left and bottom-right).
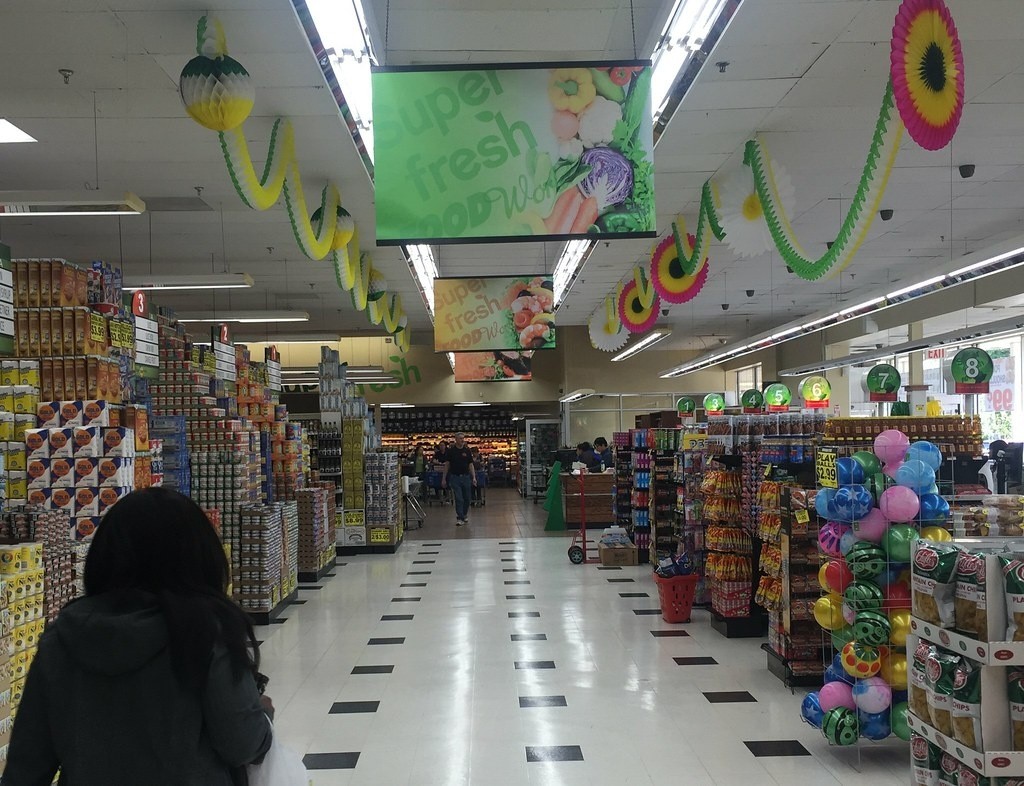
[{"x1": 598, "y1": 540, "x2": 638, "y2": 566}]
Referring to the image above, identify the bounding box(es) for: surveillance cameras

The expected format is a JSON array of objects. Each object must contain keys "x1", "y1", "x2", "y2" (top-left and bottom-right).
[{"x1": 660, "y1": 306, "x2": 671, "y2": 319}]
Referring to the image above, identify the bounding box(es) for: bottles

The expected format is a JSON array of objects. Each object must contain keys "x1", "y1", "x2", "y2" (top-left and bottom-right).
[{"x1": 289, "y1": 419, "x2": 342, "y2": 509}]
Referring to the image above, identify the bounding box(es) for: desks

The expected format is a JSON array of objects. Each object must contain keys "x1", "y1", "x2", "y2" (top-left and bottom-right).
[{"x1": 560, "y1": 473, "x2": 613, "y2": 528}]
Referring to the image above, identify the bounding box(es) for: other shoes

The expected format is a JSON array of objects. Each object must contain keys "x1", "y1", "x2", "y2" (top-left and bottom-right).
[{"x1": 471, "y1": 502, "x2": 481, "y2": 506}]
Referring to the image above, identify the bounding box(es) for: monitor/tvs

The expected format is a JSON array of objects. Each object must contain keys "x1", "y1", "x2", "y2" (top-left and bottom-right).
[{"x1": 557, "y1": 450, "x2": 577, "y2": 471}]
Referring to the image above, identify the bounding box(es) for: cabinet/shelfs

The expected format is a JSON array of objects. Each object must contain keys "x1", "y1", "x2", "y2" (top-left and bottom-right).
[{"x1": 612, "y1": 405, "x2": 988, "y2": 688}]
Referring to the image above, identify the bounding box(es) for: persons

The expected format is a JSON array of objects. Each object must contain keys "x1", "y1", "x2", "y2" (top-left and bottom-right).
[
  {"x1": 432, "y1": 440, "x2": 452, "y2": 506},
  {"x1": 407, "y1": 445, "x2": 429, "y2": 504},
  {"x1": 580, "y1": 442, "x2": 603, "y2": 473},
  {"x1": 593, "y1": 436, "x2": 616, "y2": 471},
  {"x1": 1, "y1": 487, "x2": 278, "y2": 786},
  {"x1": 987, "y1": 440, "x2": 1008, "y2": 494},
  {"x1": 575, "y1": 444, "x2": 587, "y2": 463},
  {"x1": 470, "y1": 445, "x2": 485, "y2": 508},
  {"x1": 441, "y1": 432, "x2": 477, "y2": 526},
  {"x1": 430, "y1": 445, "x2": 440, "y2": 471}
]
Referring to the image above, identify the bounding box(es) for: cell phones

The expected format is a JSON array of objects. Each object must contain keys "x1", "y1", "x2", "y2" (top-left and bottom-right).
[{"x1": 254, "y1": 671, "x2": 270, "y2": 697}]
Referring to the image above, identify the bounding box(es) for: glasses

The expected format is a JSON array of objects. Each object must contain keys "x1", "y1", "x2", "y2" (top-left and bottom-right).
[{"x1": 596, "y1": 445, "x2": 599, "y2": 447}]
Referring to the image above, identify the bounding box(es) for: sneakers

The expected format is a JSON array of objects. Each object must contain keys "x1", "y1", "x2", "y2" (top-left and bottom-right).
[
  {"x1": 456, "y1": 521, "x2": 465, "y2": 526},
  {"x1": 464, "y1": 518, "x2": 469, "y2": 522}
]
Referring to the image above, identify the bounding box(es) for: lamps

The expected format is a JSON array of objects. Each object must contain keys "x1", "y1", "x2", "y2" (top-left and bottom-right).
[
  {"x1": 660, "y1": 140, "x2": 1023, "y2": 381},
  {"x1": 280, "y1": 341, "x2": 399, "y2": 385},
  {"x1": 610, "y1": 328, "x2": 672, "y2": 363},
  {"x1": 511, "y1": 402, "x2": 524, "y2": 421},
  {"x1": 288, "y1": 0, "x2": 455, "y2": 380},
  {"x1": 176, "y1": 253, "x2": 310, "y2": 322},
  {"x1": 119, "y1": 201, "x2": 253, "y2": 289},
  {"x1": 231, "y1": 290, "x2": 341, "y2": 343},
  {"x1": 559, "y1": 389, "x2": 594, "y2": 402},
  {"x1": 0, "y1": 94, "x2": 145, "y2": 217},
  {"x1": 554, "y1": 0, "x2": 742, "y2": 307}
]
